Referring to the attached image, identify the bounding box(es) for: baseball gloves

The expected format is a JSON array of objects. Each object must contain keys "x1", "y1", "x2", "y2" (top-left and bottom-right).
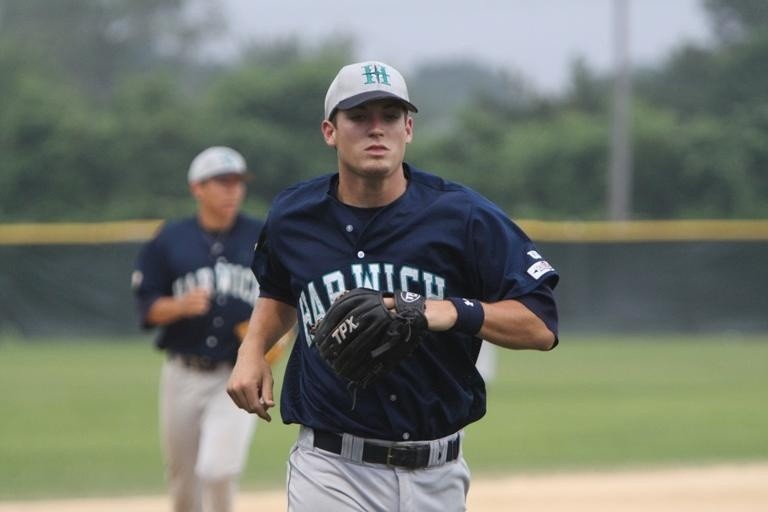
[
  {"x1": 232, "y1": 312, "x2": 294, "y2": 363},
  {"x1": 306, "y1": 288, "x2": 428, "y2": 390}
]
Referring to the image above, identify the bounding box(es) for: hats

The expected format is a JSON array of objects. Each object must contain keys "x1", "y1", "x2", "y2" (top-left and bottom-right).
[
  {"x1": 187, "y1": 146, "x2": 257, "y2": 188},
  {"x1": 323, "y1": 62, "x2": 419, "y2": 121}
]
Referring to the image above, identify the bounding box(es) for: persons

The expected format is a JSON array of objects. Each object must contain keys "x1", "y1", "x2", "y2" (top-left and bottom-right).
[
  {"x1": 228, "y1": 63, "x2": 559, "y2": 512},
  {"x1": 130, "y1": 147, "x2": 296, "y2": 512}
]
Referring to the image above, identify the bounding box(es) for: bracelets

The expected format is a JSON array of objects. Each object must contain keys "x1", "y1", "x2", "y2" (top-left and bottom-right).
[{"x1": 449, "y1": 297, "x2": 487, "y2": 334}]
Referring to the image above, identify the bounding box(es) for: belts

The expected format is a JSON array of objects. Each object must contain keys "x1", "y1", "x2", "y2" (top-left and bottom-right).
[{"x1": 313, "y1": 428, "x2": 459, "y2": 470}]
